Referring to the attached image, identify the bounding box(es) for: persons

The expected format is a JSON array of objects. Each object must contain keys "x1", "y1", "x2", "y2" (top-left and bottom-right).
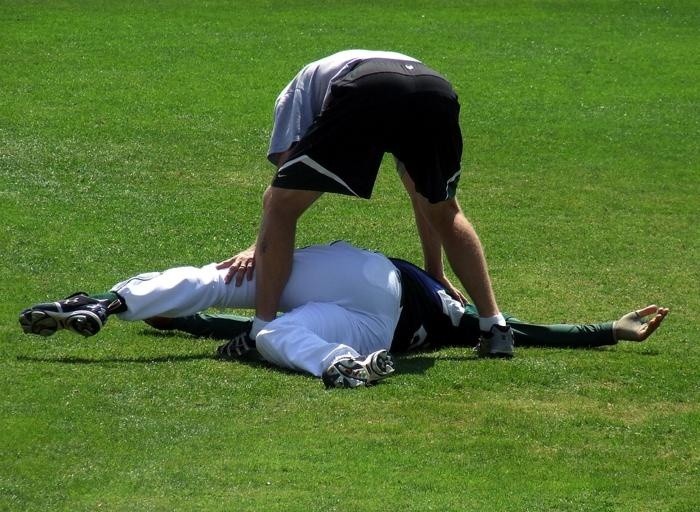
[
  {"x1": 213, "y1": 49, "x2": 514, "y2": 358},
  {"x1": 18, "y1": 241, "x2": 670, "y2": 390}
]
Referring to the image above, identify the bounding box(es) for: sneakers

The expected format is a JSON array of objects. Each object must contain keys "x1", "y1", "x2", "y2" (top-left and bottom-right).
[
  {"x1": 19, "y1": 291, "x2": 122, "y2": 338},
  {"x1": 476, "y1": 324, "x2": 513, "y2": 358},
  {"x1": 217, "y1": 330, "x2": 259, "y2": 358},
  {"x1": 321, "y1": 348, "x2": 396, "y2": 387}
]
